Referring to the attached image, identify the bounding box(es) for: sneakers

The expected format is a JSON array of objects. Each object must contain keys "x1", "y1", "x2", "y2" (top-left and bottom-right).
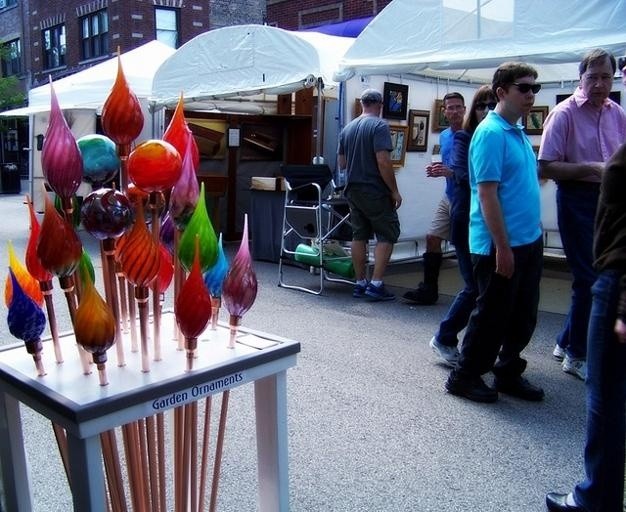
[
  {"x1": 553, "y1": 344, "x2": 565, "y2": 359},
  {"x1": 365, "y1": 282, "x2": 396, "y2": 300},
  {"x1": 562, "y1": 352, "x2": 588, "y2": 380},
  {"x1": 352, "y1": 284, "x2": 366, "y2": 298},
  {"x1": 428, "y1": 335, "x2": 460, "y2": 368}
]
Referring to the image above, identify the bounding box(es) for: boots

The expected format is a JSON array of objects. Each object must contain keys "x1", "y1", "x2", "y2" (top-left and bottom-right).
[{"x1": 402, "y1": 252, "x2": 442, "y2": 305}]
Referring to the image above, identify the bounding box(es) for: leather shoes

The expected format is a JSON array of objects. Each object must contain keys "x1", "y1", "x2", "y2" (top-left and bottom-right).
[
  {"x1": 445, "y1": 372, "x2": 498, "y2": 404},
  {"x1": 492, "y1": 375, "x2": 545, "y2": 402},
  {"x1": 546, "y1": 493, "x2": 583, "y2": 512}
]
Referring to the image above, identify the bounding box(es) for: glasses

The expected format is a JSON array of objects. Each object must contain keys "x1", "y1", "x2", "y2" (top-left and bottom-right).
[
  {"x1": 475, "y1": 102, "x2": 497, "y2": 111},
  {"x1": 510, "y1": 82, "x2": 541, "y2": 94}
]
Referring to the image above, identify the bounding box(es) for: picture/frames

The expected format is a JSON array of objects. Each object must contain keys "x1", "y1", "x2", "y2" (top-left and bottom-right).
[
  {"x1": 404, "y1": 109, "x2": 430, "y2": 153},
  {"x1": 432, "y1": 99, "x2": 453, "y2": 132},
  {"x1": 386, "y1": 124, "x2": 409, "y2": 169},
  {"x1": 382, "y1": 81, "x2": 410, "y2": 121},
  {"x1": 521, "y1": 106, "x2": 550, "y2": 136}
]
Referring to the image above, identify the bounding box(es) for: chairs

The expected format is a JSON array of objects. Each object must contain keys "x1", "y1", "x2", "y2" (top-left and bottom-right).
[{"x1": 277, "y1": 163, "x2": 371, "y2": 295}]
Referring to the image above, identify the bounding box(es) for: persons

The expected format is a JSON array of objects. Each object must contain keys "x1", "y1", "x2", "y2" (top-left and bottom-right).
[
  {"x1": 444, "y1": 60, "x2": 545, "y2": 404},
  {"x1": 546, "y1": 141, "x2": 626, "y2": 512},
  {"x1": 429, "y1": 85, "x2": 499, "y2": 368},
  {"x1": 336, "y1": 89, "x2": 402, "y2": 300},
  {"x1": 401, "y1": 92, "x2": 466, "y2": 306},
  {"x1": 536, "y1": 48, "x2": 626, "y2": 382}
]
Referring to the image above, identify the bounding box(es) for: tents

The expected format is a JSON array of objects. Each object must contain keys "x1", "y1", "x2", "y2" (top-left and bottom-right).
[
  {"x1": 331, "y1": 0, "x2": 626, "y2": 270},
  {"x1": 147, "y1": 24, "x2": 358, "y2": 243},
  {"x1": 0, "y1": 39, "x2": 177, "y2": 213}
]
